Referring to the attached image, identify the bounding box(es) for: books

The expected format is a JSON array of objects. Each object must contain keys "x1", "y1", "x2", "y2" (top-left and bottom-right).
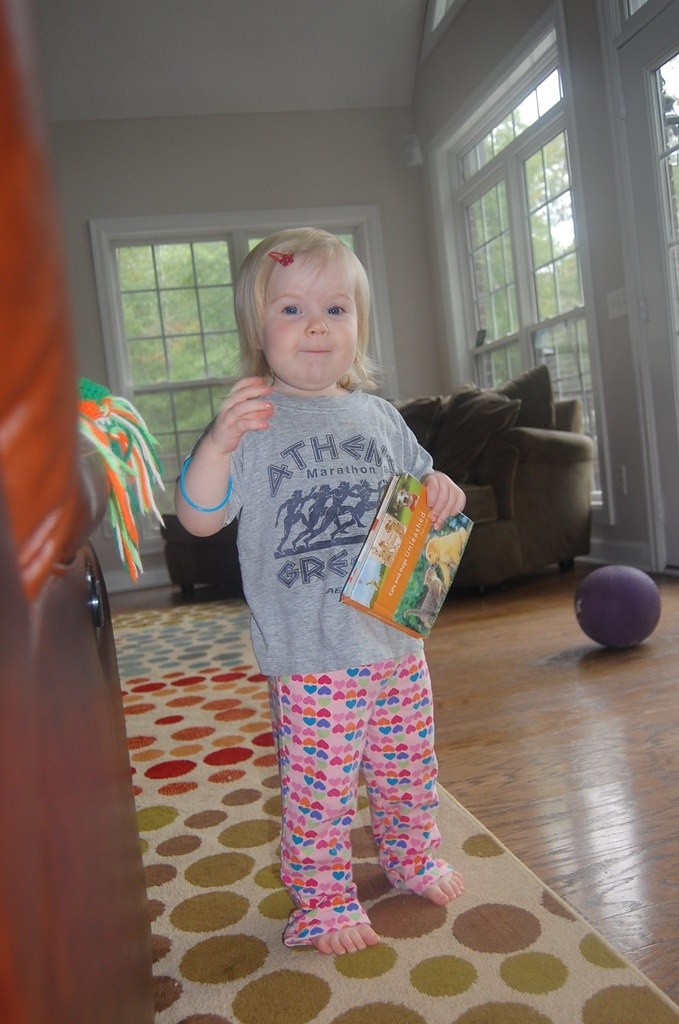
[{"x1": 336, "y1": 471, "x2": 476, "y2": 640}]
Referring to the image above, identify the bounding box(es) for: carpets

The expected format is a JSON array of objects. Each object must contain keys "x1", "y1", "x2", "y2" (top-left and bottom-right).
[{"x1": 113, "y1": 596, "x2": 679, "y2": 1023}]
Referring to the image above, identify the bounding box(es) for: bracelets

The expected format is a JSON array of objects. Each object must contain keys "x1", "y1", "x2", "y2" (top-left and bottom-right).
[{"x1": 179, "y1": 456, "x2": 234, "y2": 513}]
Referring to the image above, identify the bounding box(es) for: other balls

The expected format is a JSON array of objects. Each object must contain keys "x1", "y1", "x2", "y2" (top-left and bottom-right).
[{"x1": 573, "y1": 564, "x2": 662, "y2": 651}]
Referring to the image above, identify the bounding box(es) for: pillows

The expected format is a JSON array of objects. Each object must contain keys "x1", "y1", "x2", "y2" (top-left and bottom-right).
[
  {"x1": 423, "y1": 380, "x2": 525, "y2": 484},
  {"x1": 382, "y1": 393, "x2": 443, "y2": 447}
]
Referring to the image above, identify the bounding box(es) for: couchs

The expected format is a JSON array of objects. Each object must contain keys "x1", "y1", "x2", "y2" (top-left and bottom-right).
[{"x1": 161, "y1": 363, "x2": 595, "y2": 597}]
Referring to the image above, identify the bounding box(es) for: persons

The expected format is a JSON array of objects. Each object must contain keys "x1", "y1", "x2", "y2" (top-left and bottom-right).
[{"x1": 171, "y1": 225, "x2": 471, "y2": 956}]
